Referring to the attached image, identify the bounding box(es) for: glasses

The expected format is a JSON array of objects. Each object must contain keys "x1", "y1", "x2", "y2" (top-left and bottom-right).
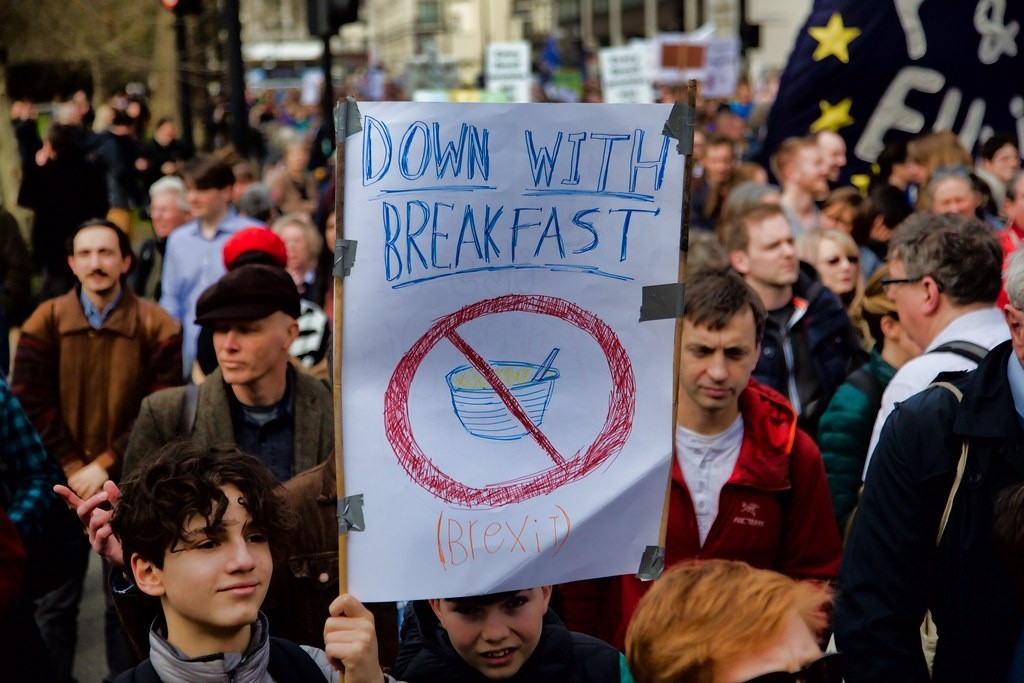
[{"x1": 880, "y1": 274, "x2": 928, "y2": 288}]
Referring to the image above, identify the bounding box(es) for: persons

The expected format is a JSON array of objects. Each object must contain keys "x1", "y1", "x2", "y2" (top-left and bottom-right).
[{"x1": 0, "y1": 28, "x2": 1024, "y2": 682}]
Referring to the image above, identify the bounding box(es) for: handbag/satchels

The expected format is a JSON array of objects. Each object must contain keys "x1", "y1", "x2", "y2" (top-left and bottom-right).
[{"x1": 825, "y1": 610, "x2": 940, "y2": 674}]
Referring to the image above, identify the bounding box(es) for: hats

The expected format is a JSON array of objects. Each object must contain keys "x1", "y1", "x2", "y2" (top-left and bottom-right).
[
  {"x1": 224, "y1": 226, "x2": 289, "y2": 268},
  {"x1": 194, "y1": 264, "x2": 301, "y2": 322}
]
